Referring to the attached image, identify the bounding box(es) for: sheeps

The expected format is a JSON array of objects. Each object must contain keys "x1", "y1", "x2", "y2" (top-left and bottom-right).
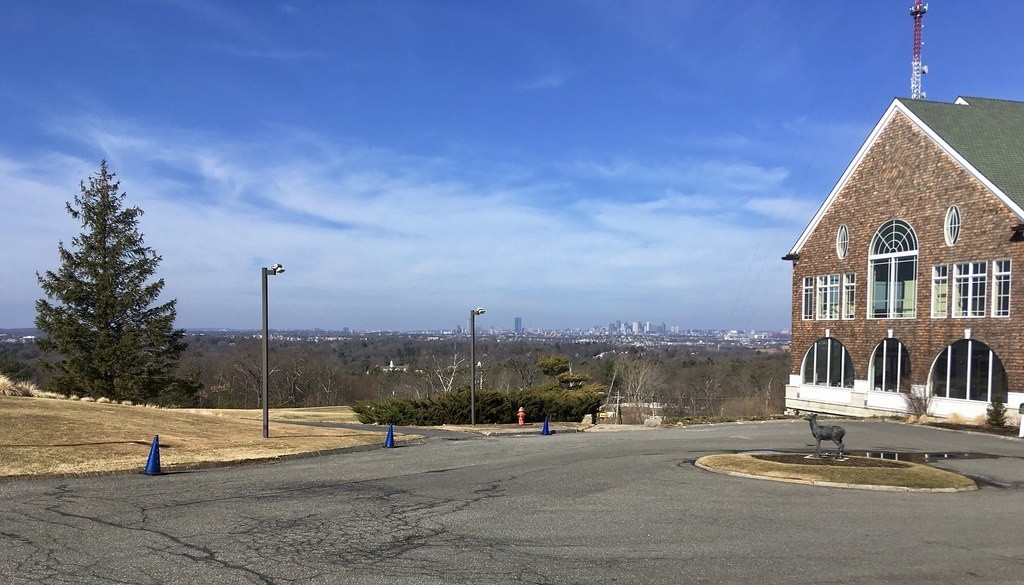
[{"x1": 802, "y1": 412, "x2": 846, "y2": 459}]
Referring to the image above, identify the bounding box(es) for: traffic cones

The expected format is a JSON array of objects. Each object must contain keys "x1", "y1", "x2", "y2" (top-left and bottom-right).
[
  {"x1": 140, "y1": 434, "x2": 165, "y2": 476},
  {"x1": 541, "y1": 416, "x2": 550, "y2": 435},
  {"x1": 383, "y1": 424, "x2": 394, "y2": 448}
]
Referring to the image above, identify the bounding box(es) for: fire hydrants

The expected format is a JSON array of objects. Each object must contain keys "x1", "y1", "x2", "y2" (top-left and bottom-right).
[{"x1": 517, "y1": 406, "x2": 526, "y2": 425}]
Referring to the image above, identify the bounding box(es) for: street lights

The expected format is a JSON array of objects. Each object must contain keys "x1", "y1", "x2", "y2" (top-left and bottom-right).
[
  {"x1": 469, "y1": 307, "x2": 486, "y2": 425},
  {"x1": 259, "y1": 263, "x2": 286, "y2": 438}
]
